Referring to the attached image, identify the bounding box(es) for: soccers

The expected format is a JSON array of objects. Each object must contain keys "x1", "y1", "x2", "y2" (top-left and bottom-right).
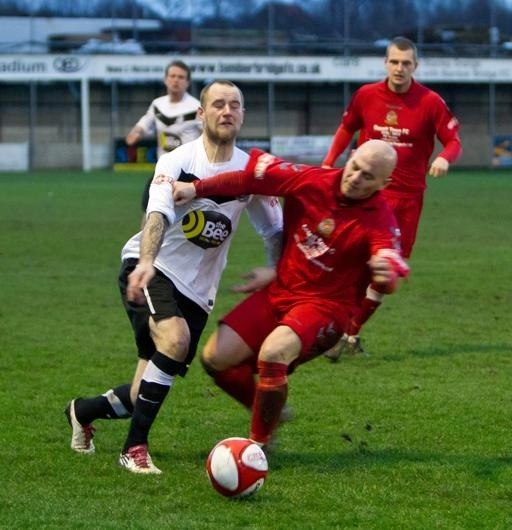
[{"x1": 207, "y1": 437, "x2": 267, "y2": 496}]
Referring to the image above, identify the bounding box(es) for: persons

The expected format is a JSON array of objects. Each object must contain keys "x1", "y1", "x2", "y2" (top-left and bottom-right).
[
  {"x1": 171, "y1": 138, "x2": 410, "y2": 454},
  {"x1": 125, "y1": 62, "x2": 203, "y2": 231},
  {"x1": 321, "y1": 35, "x2": 463, "y2": 364},
  {"x1": 64, "y1": 79, "x2": 285, "y2": 476}
]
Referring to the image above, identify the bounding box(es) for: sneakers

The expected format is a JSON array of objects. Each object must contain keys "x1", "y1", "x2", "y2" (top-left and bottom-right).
[
  {"x1": 64, "y1": 397, "x2": 96, "y2": 456},
  {"x1": 347, "y1": 334, "x2": 364, "y2": 354},
  {"x1": 324, "y1": 333, "x2": 348, "y2": 361},
  {"x1": 118, "y1": 442, "x2": 162, "y2": 475}
]
